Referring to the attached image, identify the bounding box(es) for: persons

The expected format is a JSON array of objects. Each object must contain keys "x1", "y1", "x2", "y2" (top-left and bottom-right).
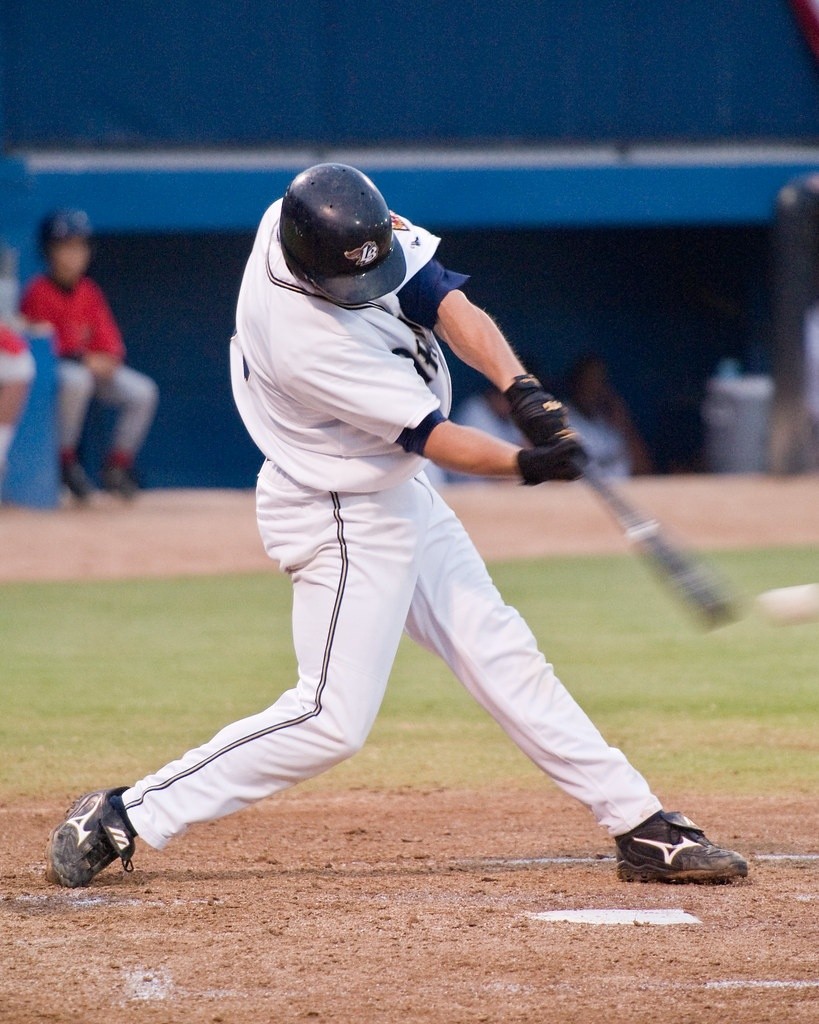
[
  {"x1": 44, "y1": 161, "x2": 749, "y2": 890},
  {"x1": 0, "y1": 240, "x2": 35, "y2": 472},
  {"x1": 445, "y1": 358, "x2": 650, "y2": 483},
  {"x1": 771, "y1": 174, "x2": 819, "y2": 472},
  {"x1": 19, "y1": 212, "x2": 159, "y2": 500}
]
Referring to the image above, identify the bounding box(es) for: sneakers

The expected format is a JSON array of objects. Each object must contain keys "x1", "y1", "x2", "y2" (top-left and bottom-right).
[
  {"x1": 44, "y1": 786, "x2": 135, "y2": 888},
  {"x1": 615, "y1": 812, "x2": 748, "y2": 884}
]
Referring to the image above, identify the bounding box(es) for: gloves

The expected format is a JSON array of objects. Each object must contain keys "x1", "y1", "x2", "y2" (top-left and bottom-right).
[
  {"x1": 502, "y1": 373, "x2": 571, "y2": 446},
  {"x1": 517, "y1": 428, "x2": 592, "y2": 485}
]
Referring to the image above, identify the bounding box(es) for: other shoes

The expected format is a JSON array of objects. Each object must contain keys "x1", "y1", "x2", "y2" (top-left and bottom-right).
[
  {"x1": 99, "y1": 465, "x2": 138, "y2": 498},
  {"x1": 63, "y1": 459, "x2": 94, "y2": 497}
]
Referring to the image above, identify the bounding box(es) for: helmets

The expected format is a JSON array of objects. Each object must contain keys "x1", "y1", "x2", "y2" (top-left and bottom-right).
[
  {"x1": 41, "y1": 208, "x2": 90, "y2": 241},
  {"x1": 280, "y1": 163, "x2": 407, "y2": 305}
]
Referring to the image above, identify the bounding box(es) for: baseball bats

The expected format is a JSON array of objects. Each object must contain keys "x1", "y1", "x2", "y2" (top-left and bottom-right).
[{"x1": 552, "y1": 436, "x2": 741, "y2": 629}]
